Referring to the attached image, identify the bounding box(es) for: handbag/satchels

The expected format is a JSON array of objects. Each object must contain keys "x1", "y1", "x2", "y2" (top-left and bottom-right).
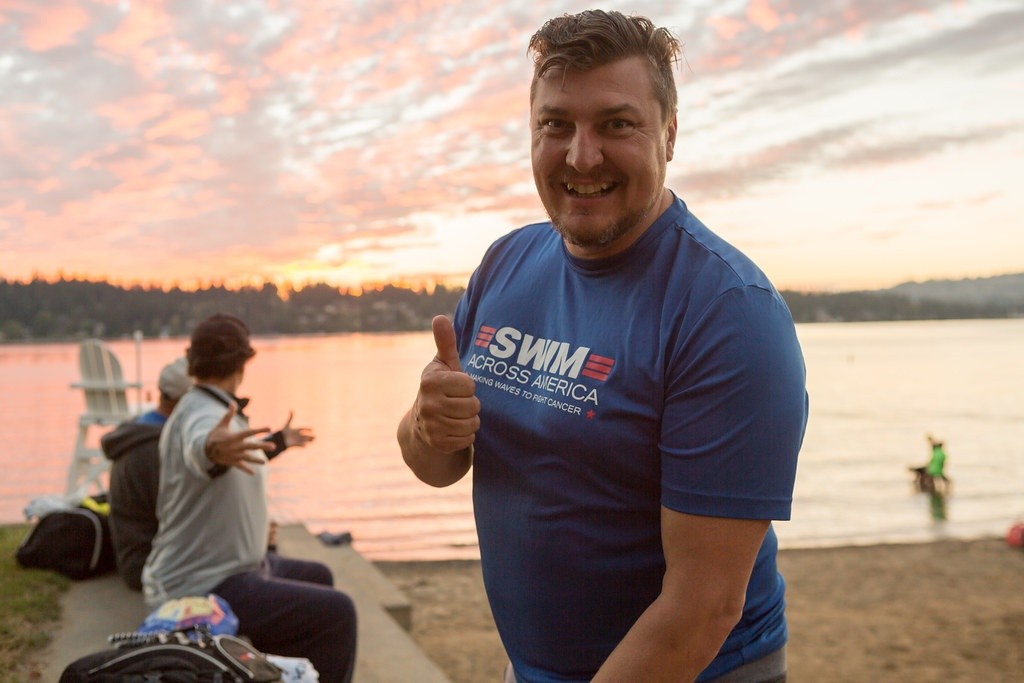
[{"x1": 18, "y1": 494, "x2": 114, "y2": 578}]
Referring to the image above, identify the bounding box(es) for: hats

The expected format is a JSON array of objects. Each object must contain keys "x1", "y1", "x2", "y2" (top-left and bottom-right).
[
  {"x1": 158, "y1": 358, "x2": 196, "y2": 400},
  {"x1": 191, "y1": 314, "x2": 255, "y2": 358}
]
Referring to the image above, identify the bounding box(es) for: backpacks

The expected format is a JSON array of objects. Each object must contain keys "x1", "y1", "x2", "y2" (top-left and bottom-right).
[{"x1": 59, "y1": 623, "x2": 283, "y2": 683}]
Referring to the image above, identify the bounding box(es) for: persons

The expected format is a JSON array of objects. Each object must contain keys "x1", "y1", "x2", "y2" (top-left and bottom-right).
[
  {"x1": 97, "y1": 355, "x2": 314, "y2": 591},
  {"x1": 924, "y1": 435, "x2": 950, "y2": 490},
  {"x1": 141, "y1": 312, "x2": 359, "y2": 682},
  {"x1": 397, "y1": 10, "x2": 810, "y2": 683},
  {"x1": 909, "y1": 467, "x2": 939, "y2": 490}
]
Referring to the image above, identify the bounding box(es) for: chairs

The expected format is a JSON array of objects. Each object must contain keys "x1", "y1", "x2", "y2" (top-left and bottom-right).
[{"x1": 65, "y1": 341, "x2": 152, "y2": 503}]
